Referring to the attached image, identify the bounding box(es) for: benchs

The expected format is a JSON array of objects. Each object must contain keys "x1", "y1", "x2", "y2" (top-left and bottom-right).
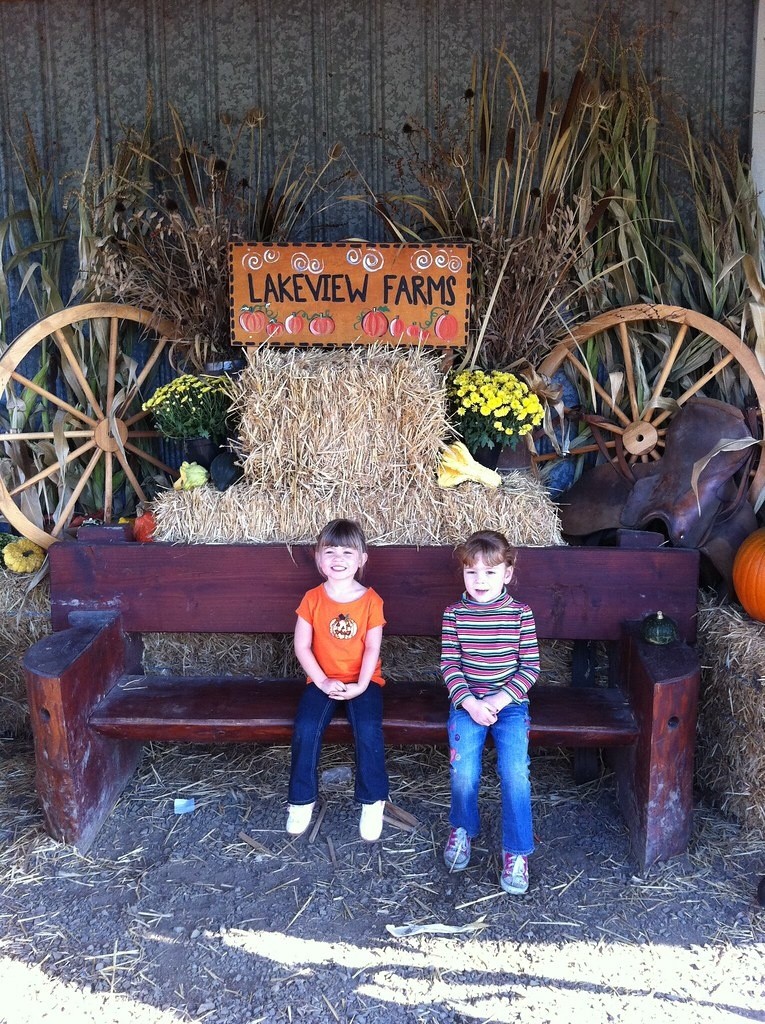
[{"x1": 23, "y1": 524, "x2": 703, "y2": 866}]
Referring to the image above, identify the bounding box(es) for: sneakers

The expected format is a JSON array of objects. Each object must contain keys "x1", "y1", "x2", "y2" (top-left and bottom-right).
[
  {"x1": 500, "y1": 849, "x2": 529, "y2": 895},
  {"x1": 443, "y1": 827, "x2": 472, "y2": 875}
]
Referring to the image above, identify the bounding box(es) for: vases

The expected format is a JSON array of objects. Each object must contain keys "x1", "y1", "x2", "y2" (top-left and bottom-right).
[{"x1": 475, "y1": 442, "x2": 502, "y2": 468}]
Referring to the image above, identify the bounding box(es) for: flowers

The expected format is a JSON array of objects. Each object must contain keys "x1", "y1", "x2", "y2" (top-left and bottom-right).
[
  {"x1": 456, "y1": 371, "x2": 544, "y2": 454},
  {"x1": 141, "y1": 373, "x2": 226, "y2": 436}
]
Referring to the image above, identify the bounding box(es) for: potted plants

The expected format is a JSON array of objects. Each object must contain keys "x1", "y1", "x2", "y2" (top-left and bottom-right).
[{"x1": 189, "y1": 437, "x2": 216, "y2": 471}]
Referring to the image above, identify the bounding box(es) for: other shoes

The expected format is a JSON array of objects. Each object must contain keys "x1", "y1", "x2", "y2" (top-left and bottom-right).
[
  {"x1": 286, "y1": 801, "x2": 315, "y2": 834},
  {"x1": 359, "y1": 800, "x2": 386, "y2": 842}
]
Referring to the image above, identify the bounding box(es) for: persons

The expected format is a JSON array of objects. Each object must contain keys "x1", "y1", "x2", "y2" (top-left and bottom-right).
[
  {"x1": 441, "y1": 529, "x2": 541, "y2": 895},
  {"x1": 286, "y1": 518, "x2": 389, "y2": 840}
]
